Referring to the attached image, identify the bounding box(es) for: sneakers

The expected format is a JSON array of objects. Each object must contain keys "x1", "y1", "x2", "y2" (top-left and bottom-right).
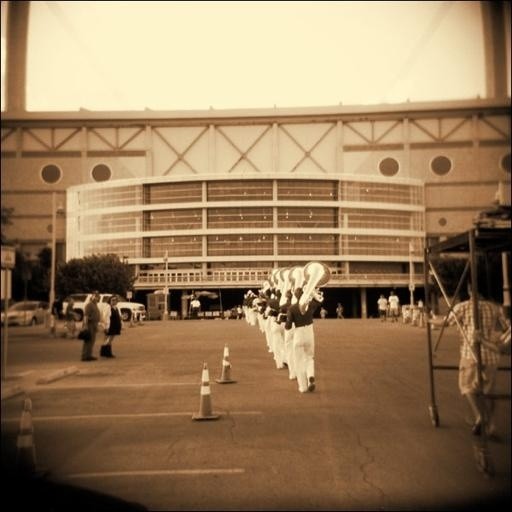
[
  {"x1": 488, "y1": 426, "x2": 502, "y2": 444},
  {"x1": 307, "y1": 376, "x2": 316, "y2": 391},
  {"x1": 472, "y1": 415, "x2": 487, "y2": 435}
]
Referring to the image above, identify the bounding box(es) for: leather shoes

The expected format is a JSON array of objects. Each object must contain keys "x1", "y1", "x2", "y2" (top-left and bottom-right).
[{"x1": 82, "y1": 356, "x2": 98, "y2": 361}]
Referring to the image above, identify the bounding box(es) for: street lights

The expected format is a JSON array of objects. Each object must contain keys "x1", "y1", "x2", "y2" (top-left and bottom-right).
[
  {"x1": 163, "y1": 249, "x2": 168, "y2": 313},
  {"x1": 408, "y1": 242, "x2": 416, "y2": 306},
  {"x1": 122, "y1": 255, "x2": 129, "y2": 264}
]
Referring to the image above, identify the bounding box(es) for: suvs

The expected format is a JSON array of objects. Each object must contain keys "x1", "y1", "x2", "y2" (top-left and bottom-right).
[{"x1": 63, "y1": 292, "x2": 147, "y2": 321}]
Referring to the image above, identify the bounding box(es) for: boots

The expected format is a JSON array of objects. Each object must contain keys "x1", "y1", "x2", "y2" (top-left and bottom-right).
[
  {"x1": 100, "y1": 345, "x2": 109, "y2": 357},
  {"x1": 107, "y1": 345, "x2": 116, "y2": 358}
]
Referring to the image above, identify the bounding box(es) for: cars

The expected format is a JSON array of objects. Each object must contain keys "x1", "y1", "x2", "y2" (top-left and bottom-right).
[{"x1": 1, "y1": 300, "x2": 59, "y2": 327}]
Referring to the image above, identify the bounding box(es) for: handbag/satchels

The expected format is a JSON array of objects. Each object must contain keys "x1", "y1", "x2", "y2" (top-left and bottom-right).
[{"x1": 78, "y1": 329, "x2": 92, "y2": 342}]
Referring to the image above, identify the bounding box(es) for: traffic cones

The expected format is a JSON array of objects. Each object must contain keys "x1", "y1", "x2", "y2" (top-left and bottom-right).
[
  {"x1": 191, "y1": 359, "x2": 219, "y2": 420},
  {"x1": 14, "y1": 398, "x2": 43, "y2": 473},
  {"x1": 215, "y1": 344, "x2": 235, "y2": 383}
]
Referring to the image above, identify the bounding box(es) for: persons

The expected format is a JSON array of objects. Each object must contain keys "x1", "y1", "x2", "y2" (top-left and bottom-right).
[
  {"x1": 61, "y1": 298, "x2": 82, "y2": 339},
  {"x1": 319, "y1": 307, "x2": 326, "y2": 319},
  {"x1": 337, "y1": 302, "x2": 345, "y2": 319},
  {"x1": 80, "y1": 289, "x2": 102, "y2": 361},
  {"x1": 242, "y1": 286, "x2": 323, "y2": 393},
  {"x1": 388, "y1": 290, "x2": 400, "y2": 322},
  {"x1": 51, "y1": 293, "x2": 66, "y2": 337},
  {"x1": 422, "y1": 274, "x2": 510, "y2": 440},
  {"x1": 237, "y1": 305, "x2": 243, "y2": 320},
  {"x1": 100, "y1": 294, "x2": 126, "y2": 357},
  {"x1": 191, "y1": 296, "x2": 202, "y2": 320},
  {"x1": 375, "y1": 293, "x2": 389, "y2": 321}
]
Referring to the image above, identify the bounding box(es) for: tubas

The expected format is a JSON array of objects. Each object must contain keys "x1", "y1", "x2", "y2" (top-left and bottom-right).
[{"x1": 266, "y1": 261, "x2": 330, "y2": 314}]
